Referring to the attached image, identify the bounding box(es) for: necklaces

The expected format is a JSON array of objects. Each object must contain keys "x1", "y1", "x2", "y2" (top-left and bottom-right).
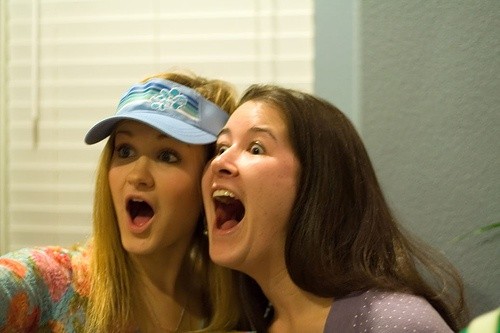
[{"x1": 173, "y1": 289, "x2": 191, "y2": 333}]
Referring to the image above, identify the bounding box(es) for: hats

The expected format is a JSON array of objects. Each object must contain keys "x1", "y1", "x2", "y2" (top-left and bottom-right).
[{"x1": 84, "y1": 78, "x2": 230, "y2": 145}]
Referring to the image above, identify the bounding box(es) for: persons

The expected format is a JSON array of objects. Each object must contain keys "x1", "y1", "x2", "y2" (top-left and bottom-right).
[
  {"x1": 0, "y1": 73, "x2": 268, "y2": 333},
  {"x1": 202, "y1": 84, "x2": 466, "y2": 333}
]
